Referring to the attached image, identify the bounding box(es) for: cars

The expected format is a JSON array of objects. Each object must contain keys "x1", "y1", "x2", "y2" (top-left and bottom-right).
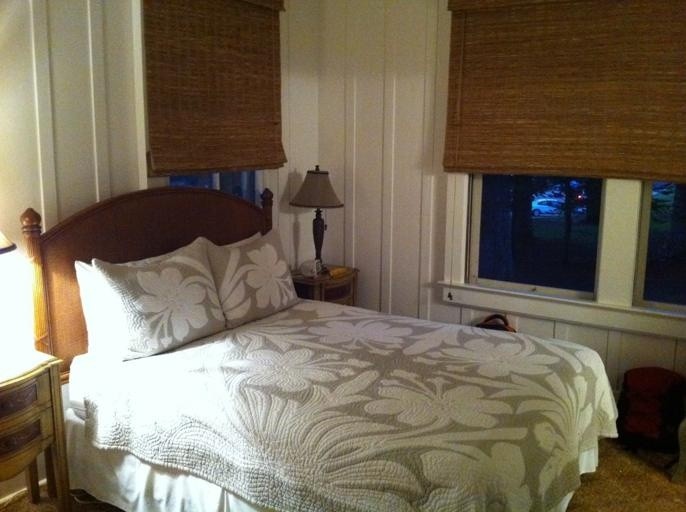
[{"x1": 527, "y1": 190, "x2": 586, "y2": 217}]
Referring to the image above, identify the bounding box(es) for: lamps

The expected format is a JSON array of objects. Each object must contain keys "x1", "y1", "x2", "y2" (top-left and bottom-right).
[{"x1": 288, "y1": 165, "x2": 347, "y2": 276}]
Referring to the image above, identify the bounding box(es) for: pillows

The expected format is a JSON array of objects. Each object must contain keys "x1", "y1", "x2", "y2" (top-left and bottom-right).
[{"x1": 71, "y1": 228, "x2": 303, "y2": 367}]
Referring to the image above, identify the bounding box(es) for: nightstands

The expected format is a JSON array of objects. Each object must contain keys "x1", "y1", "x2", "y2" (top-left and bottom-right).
[
  {"x1": 291, "y1": 262, "x2": 360, "y2": 306},
  {"x1": 1, "y1": 350, "x2": 74, "y2": 511}
]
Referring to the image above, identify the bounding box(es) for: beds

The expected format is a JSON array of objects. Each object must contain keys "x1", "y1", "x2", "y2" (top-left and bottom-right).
[{"x1": 20, "y1": 185, "x2": 622, "y2": 512}]
